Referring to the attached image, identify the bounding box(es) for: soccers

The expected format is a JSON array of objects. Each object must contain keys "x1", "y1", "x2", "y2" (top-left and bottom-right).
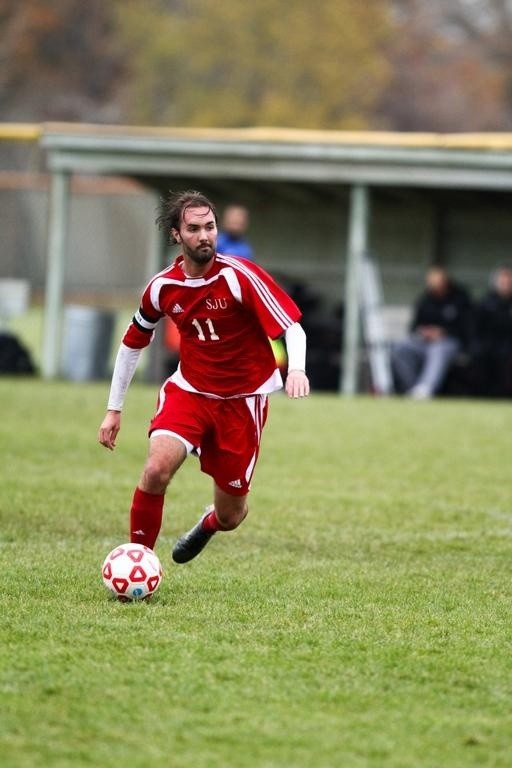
[{"x1": 101, "y1": 542, "x2": 162, "y2": 601}]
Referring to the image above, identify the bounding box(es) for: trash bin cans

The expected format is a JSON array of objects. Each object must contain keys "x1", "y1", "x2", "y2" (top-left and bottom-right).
[
  {"x1": 62, "y1": 307, "x2": 114, "y2": 382},
  {"x1": 0, "y1": 276, "x2": 31, "y2": 316}
]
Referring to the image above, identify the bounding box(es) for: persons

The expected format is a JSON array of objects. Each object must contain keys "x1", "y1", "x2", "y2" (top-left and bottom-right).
[
  {"x1": 419, "y1": 260, "x2": 512, "y2": 396},
  {"x1": 97, "y1": 186, "x2": 313, "y2": 565},
  {"x1": 404, "y1": 261, "x2": 480, "y2": 399},
  {"x1": 159, "y1": 203, "x2": 257, "y2": 382}
]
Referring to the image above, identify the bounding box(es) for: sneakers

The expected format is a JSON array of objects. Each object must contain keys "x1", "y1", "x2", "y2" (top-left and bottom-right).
[{"x1": 172, "y1": 504, "x2": 216, "y2": 564}]
